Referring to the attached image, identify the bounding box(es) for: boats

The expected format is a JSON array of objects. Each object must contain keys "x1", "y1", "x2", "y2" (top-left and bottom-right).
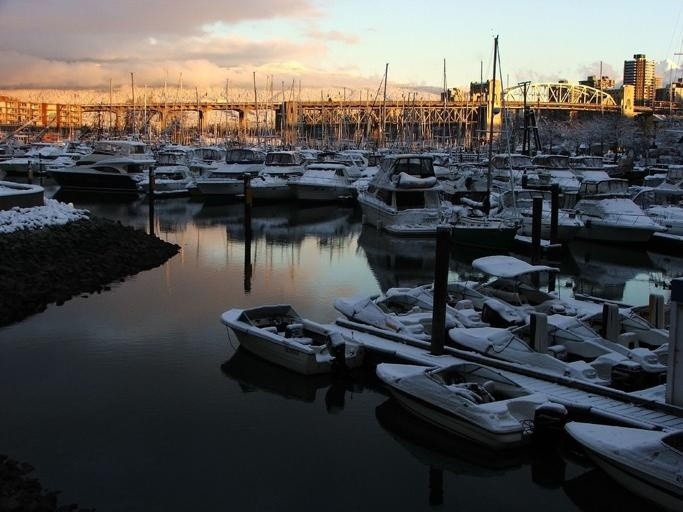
[
  {"x1": 219, "y1": 305, "x2": 358, "y2": 375},
  {"x1": 562, "y1": 417, "x2": 683, "y2": 512},
  {"x1": 375, "y1": 358, "x2": 571, "y2": 448}
]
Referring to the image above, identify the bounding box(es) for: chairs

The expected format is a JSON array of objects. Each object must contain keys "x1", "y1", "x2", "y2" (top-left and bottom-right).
[
  {"x1": 482, "y1": 381, "x2": 494, "y2": 396},
  {"x1": 260, "y1": 323, "x2": 303, "y2": 339}
]
